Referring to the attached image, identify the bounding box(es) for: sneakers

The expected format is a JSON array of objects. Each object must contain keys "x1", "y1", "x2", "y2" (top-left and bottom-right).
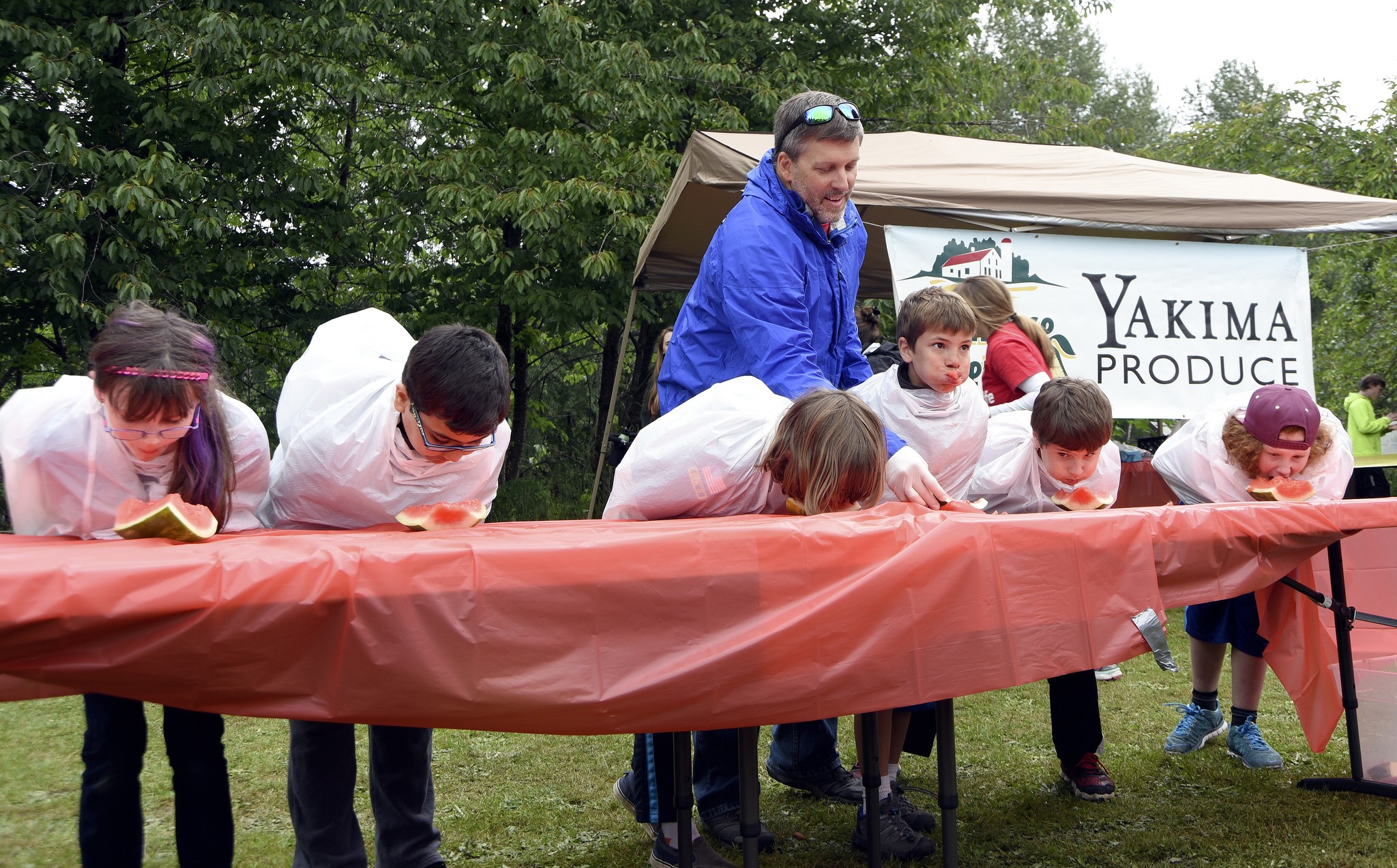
[
  {"x1": 1058, "y1": 752, "x2": 1115, "y2": 801},
  {"x1": 1227, "y1": 715, "x2": 1283, "y2": 770},
  {"x1": 853, "y1": 792, "x2": 936, "y2": 861},
  {"x1": 850, "y1": 761, "x2": 902, "y2": 781},
  {"x1": 890, "y1": 780, "x2": 938, "y2": 833},
  {"x1": 1162, "y1": 698, "x2": 1227, "y2": 754}
]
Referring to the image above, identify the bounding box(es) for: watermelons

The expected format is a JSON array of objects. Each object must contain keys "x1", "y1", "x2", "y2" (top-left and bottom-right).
[
  {"x1": 1244, "y1": 475, "x2": 1316, "y2": 502},
  {"x1": 393, "y1": 499, "x2": 487, "y2": 531},
  {"x1": 1051, "y1": 486, "x2": 1114, "y2": 511},
  {"x1": 115, "y1": 493, "x2": 219, "y2": 541}
]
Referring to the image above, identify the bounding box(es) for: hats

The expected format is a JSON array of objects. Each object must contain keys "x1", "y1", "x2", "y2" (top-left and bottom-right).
[{"x1": 1234, "y1": 382, "x2": 1321, "y2": 451}]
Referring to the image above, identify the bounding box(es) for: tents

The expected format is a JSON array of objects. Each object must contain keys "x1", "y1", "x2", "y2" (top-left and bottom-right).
[{"x1": 584, "y1": 129, "x2": 1397, "y2": 518}]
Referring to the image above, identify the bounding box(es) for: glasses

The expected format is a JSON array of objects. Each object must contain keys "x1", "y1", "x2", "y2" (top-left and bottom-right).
[
  {"x1": 778, "y1": 101, "x2": 860, "y2": 150},
  {"x1": 99, "y1": 388, "x2": 202, "y2": 439},
  {"x1": 406, "y1": 388, "x2": 495, "y2": 452}
]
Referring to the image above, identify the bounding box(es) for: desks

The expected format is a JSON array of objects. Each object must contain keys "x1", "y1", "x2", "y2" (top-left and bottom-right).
[
  {"x1": 1113, "y1": 459, "x2": 1180, "y2": 507},
  {"x1": 0, "y1": 499, "x2": 1397, "y2": 868}
]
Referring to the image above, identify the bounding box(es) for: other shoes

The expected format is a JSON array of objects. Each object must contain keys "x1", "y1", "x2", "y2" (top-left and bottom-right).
[
  {"x1": 649, "y1": 834, "x2": 737, "y2": 868},
  {"x1": 613, "y1": 770, "x2": 660, "y2": 841},
  {"x1": 765, "y1": 765, "x2": 863, "y2": 805},
  {"x1": 698, "y1": 807, "x2": 774, "y2": 851},
  {"x1": 1094, "y1": 663, "x2": 1122, "y2": 680}
]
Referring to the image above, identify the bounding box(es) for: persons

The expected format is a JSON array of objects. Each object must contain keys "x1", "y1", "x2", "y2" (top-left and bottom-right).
[
  {"x1": 613, "y1": 94, "x2": 954, "y2": 840},
  {"x1": 602, "y1": 376, "x2": 886, "y2": 868},
  {"x1": 1343, "y1": 374, "x2": 1397, "y2": 499},
  {"x1": 640, "y1": 326, "x2": 674, "y2": 427},
  {"x1": 257, "y1": 322, "x2": 511, "y2": 868},
  {"x1": 0, "y1": 304, "x2": 270, "y2": 868},
  {"x1": 848, "y1": 280, "x2": 1122, "y2": 859},
  {"x1": 1151, "y1": 385, "x2": 1355, "y2": 770}
]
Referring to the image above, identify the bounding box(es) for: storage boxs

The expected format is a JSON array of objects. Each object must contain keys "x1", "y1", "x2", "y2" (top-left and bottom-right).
[{"x1": 1135, "y1": 436, "x2": 1167, "y2": 453}]
